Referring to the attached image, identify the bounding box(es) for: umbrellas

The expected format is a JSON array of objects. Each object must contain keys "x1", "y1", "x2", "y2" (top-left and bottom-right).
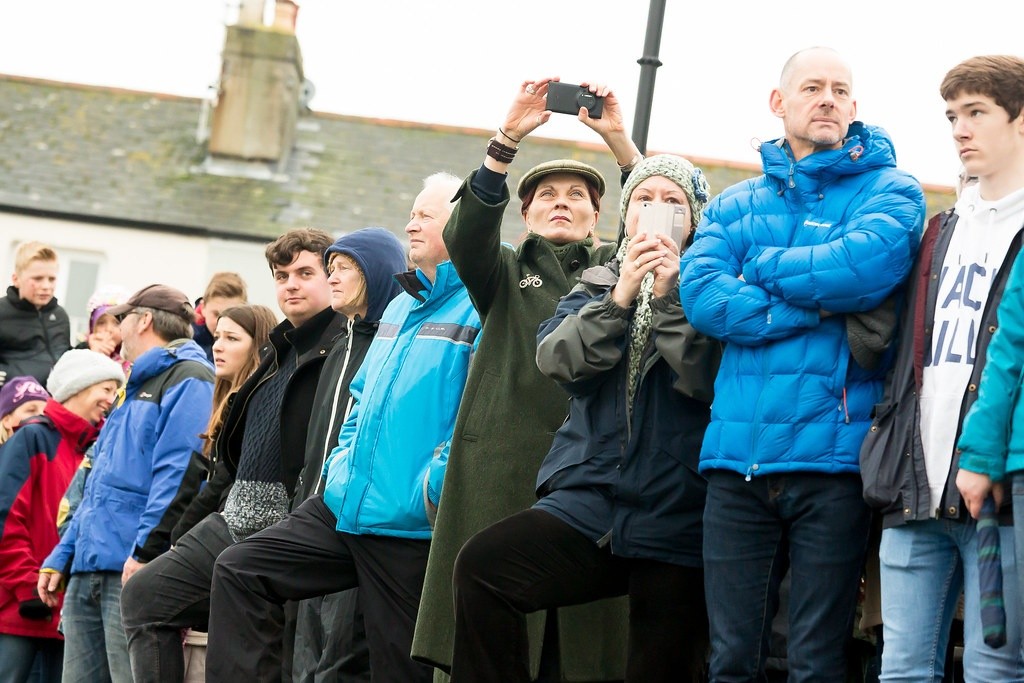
[{"x1": 973, "y1": 491, "x2": 1007, "y2": 650}]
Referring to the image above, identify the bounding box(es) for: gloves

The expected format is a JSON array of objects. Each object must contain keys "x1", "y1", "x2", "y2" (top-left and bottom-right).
[{"x1": 19, "y1": 599, "x2": 52, "y2": 621}]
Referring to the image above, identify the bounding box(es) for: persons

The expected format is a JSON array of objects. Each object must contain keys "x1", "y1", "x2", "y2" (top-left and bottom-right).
[
  {"x1": 449, "y1": 155, "x2": 726, "y2": 682},
  {"x1": 680, "y1": 48, "x2": 927, "y2": 683},
  {"x1": 956, "y1": 237, "x2": 1024, "y2": 672},
  {"x1": 848, "y1": 54, "x2": 1024, "y2": 683},
  {"x1": 409, "y1": 77, "x2": 644, "y2": 683},
  {"x1": 0, "y1": 173, "x2": 485, "y2": 683}
]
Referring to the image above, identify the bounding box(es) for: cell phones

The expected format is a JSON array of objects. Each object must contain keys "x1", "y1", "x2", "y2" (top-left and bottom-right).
[
  {"x1": 638, "y1": 201, "x2": 686, "y2": 260},
  {"x1": 545, "y1": 82, "x2": 604, "y2": 120}
]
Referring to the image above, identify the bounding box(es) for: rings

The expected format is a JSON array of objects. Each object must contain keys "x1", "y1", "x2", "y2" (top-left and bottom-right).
[
  {"x1": 634, "y1": 259, "x2": 641, "y2": 268},
  {"x1": 529, "y1": 87, "x2": 536, "y2": 94}
]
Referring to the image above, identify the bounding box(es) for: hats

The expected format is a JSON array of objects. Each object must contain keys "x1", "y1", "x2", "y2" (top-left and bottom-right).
[
  {"x1": 86, "y1": 289, "x2": 122, "y2": 335},
  {"x1": 0, "y1": 375, "x2": 49, "y2": 421},
  {"x1": 620, "y1": 154, "x2": 711, "y2": 232},
  {"x1": 45, "y1": 348, "x2": 125, "y2": 402},
  {"x1": 105, "y1": 284, "x2": 193, "y2": 325},
  {"x1": 516, "y1": 160, "x2": 606, "y2": 201}
]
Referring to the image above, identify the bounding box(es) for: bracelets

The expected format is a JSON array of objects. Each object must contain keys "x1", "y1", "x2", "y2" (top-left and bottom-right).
[
  {"x1": 498, "y1": 127, "x2": 520, "y2": 143},
  {"x1": 616, "y1": 152, "x2": 639, "y2": 170},
  {"x1": 486, "y1": 137, "x2": 520, "y2": 164}
]
